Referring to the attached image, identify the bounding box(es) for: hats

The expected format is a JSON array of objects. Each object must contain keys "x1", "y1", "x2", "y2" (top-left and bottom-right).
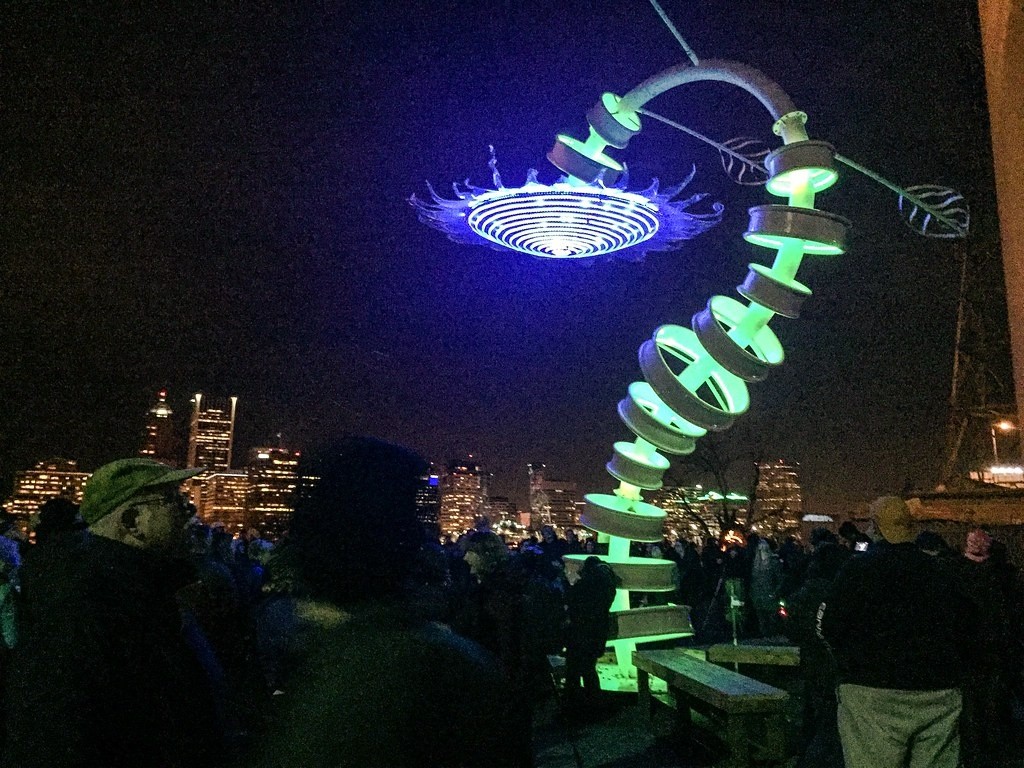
[
  {"x1": 964, "y1": 529, "x2": 991, "y2": 562},
  {"x1": 868, "y1": 495, "x2": 915, "y2": 544},
  {"x1": 80, "y1": 457, "x2": 205, "y2": 526}
]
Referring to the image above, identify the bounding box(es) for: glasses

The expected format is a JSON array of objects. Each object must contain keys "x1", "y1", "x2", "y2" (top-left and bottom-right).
[{"x1": 131, "y1": 492, "x2": 189, "y2": 507}]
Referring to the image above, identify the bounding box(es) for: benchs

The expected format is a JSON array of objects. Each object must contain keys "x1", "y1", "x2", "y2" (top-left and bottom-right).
[{"x1": 631, "y1": 643, "x2": 802, "y2": 768}]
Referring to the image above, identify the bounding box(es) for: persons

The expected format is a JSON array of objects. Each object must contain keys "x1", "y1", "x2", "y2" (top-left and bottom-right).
[
  {"x1": 630, "y1": 496, "x2": 1024, "y2": 768},
  {"x1": 0, "y1": 433, "x2": 618, "y2": 768}
]
